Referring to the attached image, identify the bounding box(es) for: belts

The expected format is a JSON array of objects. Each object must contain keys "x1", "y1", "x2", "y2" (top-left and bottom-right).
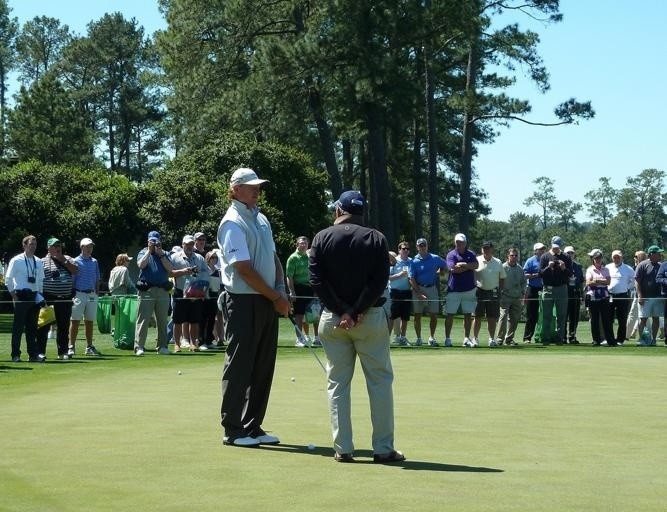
[
  {"x1": 75, "y1": 289, "x2": 94, "y2": 293},
  {"x1": 415, "y1": 283, "x2": 435, "y2": 288}
]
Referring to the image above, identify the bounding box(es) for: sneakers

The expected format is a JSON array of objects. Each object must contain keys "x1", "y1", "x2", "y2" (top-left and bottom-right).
[
  {"x1": 374, "y1": 450, "x2": 405, "y2": 463},
  {"x1": 252, "y1": 430, "x2": 280, "y2": 444},
  {"x1": 223, "y1": 432, "x2": 260, "y2": 446},
  {"x1": 392, "y1": 335, "x2": 657, "y2": 347},
  {"x1": 334, "y1": 452, "x2": 355, "y2": 462},
  {"x1": 11, "y1": 342, "x2": 224, "y2": 361},
  {"x1": 295, "y1": 335, "x2": 323, "y2": 348}
]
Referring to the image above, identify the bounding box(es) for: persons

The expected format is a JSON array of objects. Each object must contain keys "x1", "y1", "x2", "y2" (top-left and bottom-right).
[
  {"x1": 216, "y1": 167, "x2": 292, "y2": 447},
  {"x1": 308, "y1": 190, "x2": 405, "y2": 464}
]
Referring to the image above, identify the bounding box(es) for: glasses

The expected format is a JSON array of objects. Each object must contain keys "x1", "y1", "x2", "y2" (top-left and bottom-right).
[
  {"x1": 210, "y1": 256, "x2": 218, "y2": 260},
  {"x1": 401, "y1": 248, "x2": 410, "y2": 250},
  {"x1": 192, "y1": 268, "x2": 198, "y2": 277}
]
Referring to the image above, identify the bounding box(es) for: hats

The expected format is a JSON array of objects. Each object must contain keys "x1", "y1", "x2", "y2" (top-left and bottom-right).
[
  {"x1": 334, "y1": 190, "x2": 367, "y2": 216},
  {"x1": 416, "y1": 232, "x2": 664, "y2": 259},
  {"x1": 229, "y1": 167, "x2": 270, "y2": 188},
  {"x1": 147, "y1": 231, "x2": 206, "y2": 253},
  {"x1": 47, "y1": 237, "x2": 96, "y2": 248},
  {"x1": 125, "y1": 254, "x2": 133, "y2": 261}
]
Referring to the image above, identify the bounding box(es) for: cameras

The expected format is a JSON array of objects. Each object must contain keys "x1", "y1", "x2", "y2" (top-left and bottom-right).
[
  {"x1": 552, "y1": 260, "x2": 560, "y2": 266},
  {"x1": 28, "y1": 275, "x2": 36, "y2": 283},
  {"x1": 51, "y1": 270, "x2": 60, "y2": 278},
  {"x1": 152, "y1": 240, "x2": 160, "y2": 247}
]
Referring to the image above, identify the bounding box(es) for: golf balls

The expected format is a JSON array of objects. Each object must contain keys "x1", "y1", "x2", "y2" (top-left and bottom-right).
[
  {"x1": 291, "y1": 378, "x2": 295, "y2": 381},
  {"x1": 178, "y1": 372, "x2": 181, "y2": 374},
  {"x1": 308, "y1": 445, "x2": 314, "y2": 451}
]
